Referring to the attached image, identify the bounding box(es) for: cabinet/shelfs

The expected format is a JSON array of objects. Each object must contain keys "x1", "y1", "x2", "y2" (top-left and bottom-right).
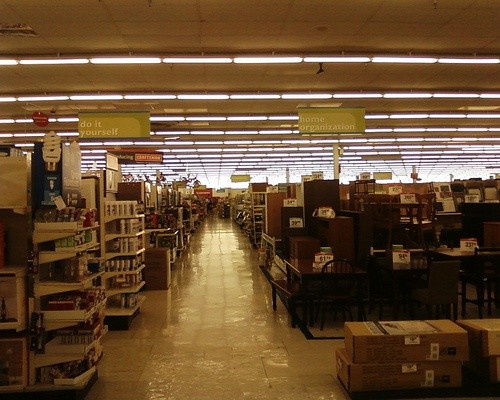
[
  {"x1": 220, "y1": 182, "x2": 304, "y2": 270},
  {"x1": 0, "y1": 144, "x2": 212, "y2": 399},
  {"x1": 304, "y1": 179, "x2": 341, "y2": 238}
]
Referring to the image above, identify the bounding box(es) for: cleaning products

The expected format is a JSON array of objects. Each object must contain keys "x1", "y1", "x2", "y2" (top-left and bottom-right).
[{"x1": 105, "y1": 200, "x2": 144, "y2": 310}]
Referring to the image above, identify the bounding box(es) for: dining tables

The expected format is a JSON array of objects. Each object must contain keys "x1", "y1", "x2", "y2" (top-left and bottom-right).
[
  {"x1": 284, "y1": 260, "x2": 367, "y2": 328},
  {"x1": 428, "y1": 248, "x2": 499, "y2": 318},
  {"x1": 361, "y1": 203, "x2": 425, "y2": 244},
  {"x1": 374, "y1": 255, "x2": 447, "y2": 320}
]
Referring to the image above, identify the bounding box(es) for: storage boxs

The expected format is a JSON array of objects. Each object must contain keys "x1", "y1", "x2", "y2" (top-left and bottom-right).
[
  {"x1": 336, "y1": 318, "x2": 500, "y2": 393},
  {"x1": 289, "y1": 236, "x2": 315, "y2": 260},
  {"x1": 146, "y1": 249, "x2": 171, "y2": 291},
  {"x1": 31, "y1": 140, "x2": 81, "y2": 206},
  {"x1": 321, "y1": 214, "x2": 353, "y2": 260}
]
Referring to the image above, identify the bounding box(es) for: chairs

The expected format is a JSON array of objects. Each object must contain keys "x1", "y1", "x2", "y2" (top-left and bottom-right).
[
  {"x1": 412, "y1": 260, "x2": 459, "y2": 321},
  {"x1": 351, "y1": 179, "x2": 441, "y2": 247},
  {"x1": 320, "y1": 259, "x2": 368, "y2": 330},
  {"x1": 462, "y1": 247, "x2": 500, "y2": 319}
]
